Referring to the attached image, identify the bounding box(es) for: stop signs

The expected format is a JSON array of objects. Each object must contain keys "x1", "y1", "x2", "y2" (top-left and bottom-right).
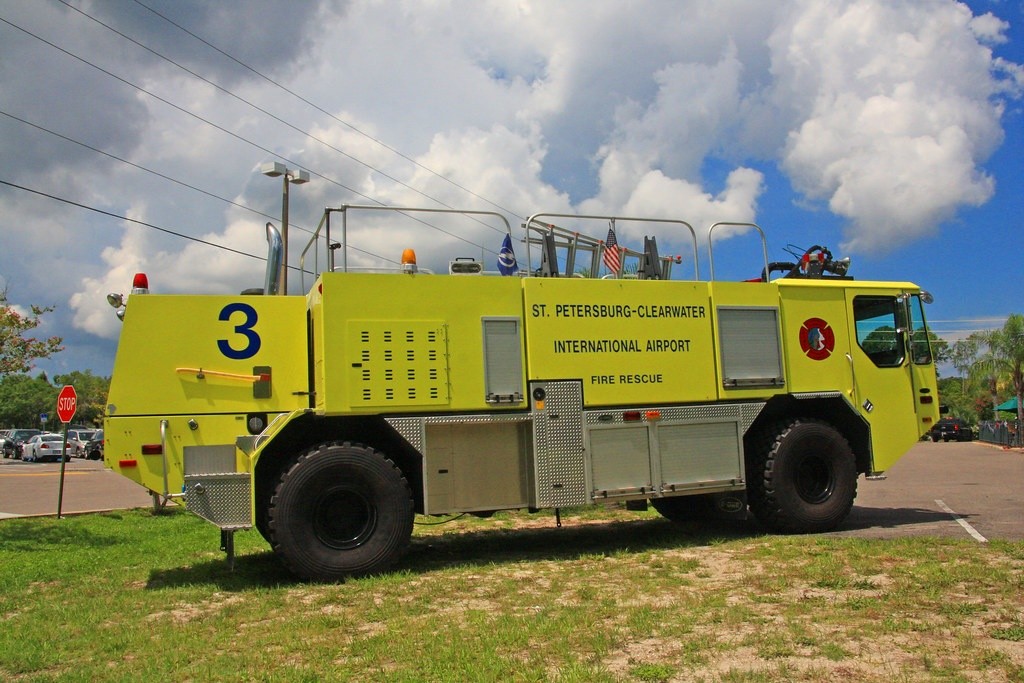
[{"x1": 58, "y1": 385, "x2": 77, "y2": 424}]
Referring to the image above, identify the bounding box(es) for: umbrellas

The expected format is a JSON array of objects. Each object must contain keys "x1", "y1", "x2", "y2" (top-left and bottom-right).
[{"x1": 992, "y1": 396, "x2": 1024, "y2": 429}]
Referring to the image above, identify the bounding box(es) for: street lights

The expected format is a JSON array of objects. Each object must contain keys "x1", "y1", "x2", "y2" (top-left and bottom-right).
[{"x1": 262, "y1": 162, "x2": 311, "y2": 296}]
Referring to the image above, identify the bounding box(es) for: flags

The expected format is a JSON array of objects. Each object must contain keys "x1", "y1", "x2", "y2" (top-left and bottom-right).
[
  {"x1": 602, "y1": 229, "x2": 620, "y2": 274},
  {"x1": 497, "y1": 233, "x2": 519, "y2": 276}
]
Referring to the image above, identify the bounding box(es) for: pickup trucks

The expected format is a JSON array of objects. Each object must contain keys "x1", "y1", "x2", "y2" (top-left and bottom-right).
[{"x1": 931, "y1": 417, "x2": 972, "y2": 442}]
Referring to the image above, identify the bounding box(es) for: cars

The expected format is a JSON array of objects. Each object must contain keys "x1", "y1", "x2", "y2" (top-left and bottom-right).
[{"x1": 0, "y1": 429, "x2": 104, "y2": 462}]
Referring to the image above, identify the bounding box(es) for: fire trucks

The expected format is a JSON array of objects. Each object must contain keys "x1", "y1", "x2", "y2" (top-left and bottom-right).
[{"x1": 104, "y1": 162, "x2": 941, "y2": 583}]
